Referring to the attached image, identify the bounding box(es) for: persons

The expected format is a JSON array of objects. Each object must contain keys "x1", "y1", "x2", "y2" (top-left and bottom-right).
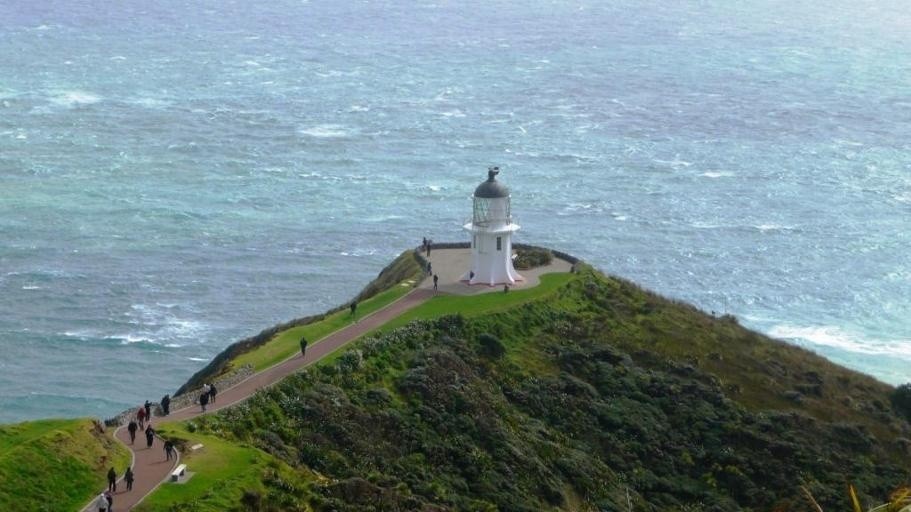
[
  {"x1": 351, "y1": 300, "x2": 357, "y2": 315},
  {"x1": 209, "y1": 384, "x2": 218, "y2": 403},
  {"x1": 137, "y1": 407, "x2": 146, "y2": 431},
  {"x1": 108, "y1": 467, "x2": 116, "y2": 492},
  {"x1": 144, "y1": 400, "x2": 153, "y2": 421},
  {"x1": 145, "y1": 424, "x2": 155, "y2": 448},
  {"x1": 426, "y1": 239, "x2": 432, "y2": 256},
  {"x1": 161, "y1": 393, "x2": 171, "y2": 413},
  {"x1": 427, "y1": 262, "x2": 433, "y2": 276},
  {"x1": 300, "y1": 336, "x2": 308, "y2": 357},
  {"x1": 105, "y1": 493, "x2": 113, "y2": 511},
  {"x1": 128, "y1": 417, "x2": 138, "y2": 444},
  {"x1": 202, "y1": 383, "x2": 210, "y2": 404},
  {"x1": 124, "y1": 466, "x2": 135, "y2": 491},
  {"x1": 163, "y1": 437, "x2": 173, "y2": 461},
  {"x1": 200, "y1": 391, "x2": 208, "y2": 412},
  {"x1": 434, "y1": 273, "x2": 439, "y2": 290},
  {"x1": 95, "y1": 493, "x2": 109, "y2": 511},
  {"x1": 422, "y1": 237, "x2": 427, "y2": 253}
]
